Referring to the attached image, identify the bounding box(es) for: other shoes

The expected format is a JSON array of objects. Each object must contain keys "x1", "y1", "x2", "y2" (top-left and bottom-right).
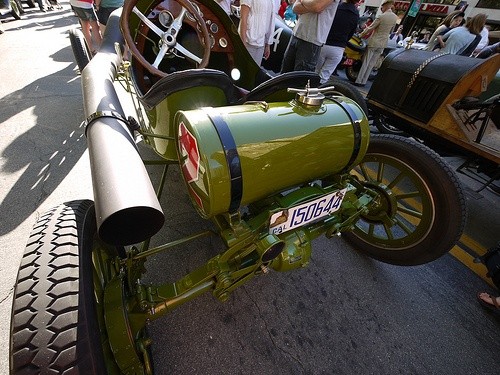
[
  {"x1": 361, "y1": 84, "x2": 365, "y2": 87},
  {"x1": 349, "y1": 81, "x2": 360, "y2": 86},
  {"x1": 40, "y1": 6, "x2": 46, "y2": 12}
]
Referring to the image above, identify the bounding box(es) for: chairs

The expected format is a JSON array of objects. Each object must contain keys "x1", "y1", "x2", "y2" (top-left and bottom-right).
[{"x1": 140, "y1": 64, "x2": 322, "y2": 162}]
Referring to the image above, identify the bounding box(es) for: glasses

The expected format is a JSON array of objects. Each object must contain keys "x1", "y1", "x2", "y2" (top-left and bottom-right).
[{"x1": 381, "y1": 8, "x2": 383, "y2": 10}]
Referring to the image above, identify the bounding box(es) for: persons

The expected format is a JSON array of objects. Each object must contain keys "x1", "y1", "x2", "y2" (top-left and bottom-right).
[
  {"x1": 70, "y1": 0, "x2": 129, "y2": 61},
  {"x1": 214, "y1": 0, "x2": 489, "y2": 86}
]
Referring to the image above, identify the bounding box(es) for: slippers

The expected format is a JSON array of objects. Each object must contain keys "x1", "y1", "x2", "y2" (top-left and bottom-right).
[{"x1": 478, "y1": 291, "x2": 500, "y2": 315}]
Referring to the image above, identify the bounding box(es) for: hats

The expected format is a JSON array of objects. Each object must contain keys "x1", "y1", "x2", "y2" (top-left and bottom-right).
[
  {"x1": 425, "y1": 31, "x2": 432, "y2": 34},
  {"x1": 382, "y1": 0, "x2": 394, "y2": 6}
]
